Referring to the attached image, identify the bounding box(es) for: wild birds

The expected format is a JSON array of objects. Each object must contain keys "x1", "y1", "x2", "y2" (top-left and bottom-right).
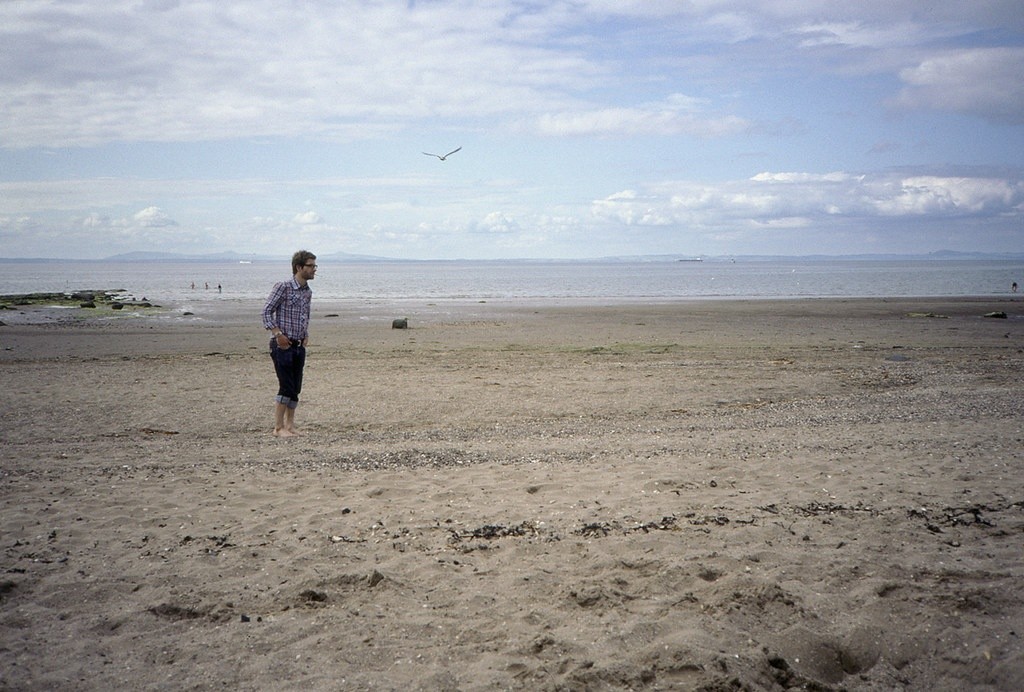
[{"x1": 422, "y1": 146, "x2": 462, "y2": 160}]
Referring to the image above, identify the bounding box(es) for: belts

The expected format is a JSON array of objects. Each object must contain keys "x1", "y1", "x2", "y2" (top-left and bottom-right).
[{"x1": 270, "y1": 337, "x2": 304, "y2": 346}]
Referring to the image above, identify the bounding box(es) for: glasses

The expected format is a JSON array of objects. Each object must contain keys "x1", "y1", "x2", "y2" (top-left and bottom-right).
[{"x1": 303, "y1": 263, "x2": 318, "y2": 270}]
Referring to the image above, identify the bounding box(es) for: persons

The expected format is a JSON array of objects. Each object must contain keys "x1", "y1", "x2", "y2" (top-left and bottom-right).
[
  {"x1": 262, "y1": 250, "x2": 318, "y2": 438},
  {"x1": 191, "y1": 282, "x2": 221, "y2": 293}
]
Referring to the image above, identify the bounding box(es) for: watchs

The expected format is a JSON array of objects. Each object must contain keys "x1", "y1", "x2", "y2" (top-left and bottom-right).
[{"x1": 275, "y1": 331, "x2": 283, "y2": 337}]
